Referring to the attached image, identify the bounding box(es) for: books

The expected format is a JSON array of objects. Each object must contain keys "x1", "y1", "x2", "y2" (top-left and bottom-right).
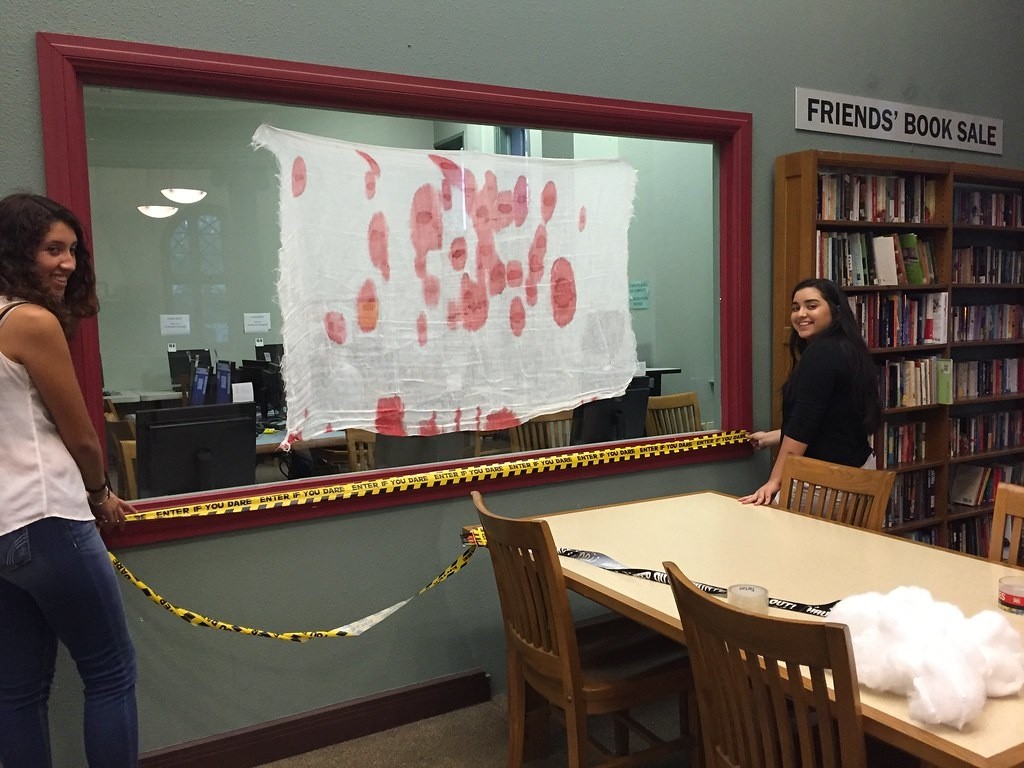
[{"x1": 818, "y1": 167, "x2": 1022, "y2": 562}]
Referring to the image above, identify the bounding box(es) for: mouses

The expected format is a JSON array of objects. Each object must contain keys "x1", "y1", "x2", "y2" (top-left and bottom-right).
[{"x1": 104, "y1": 391, "x2": 111, "y2": 396}]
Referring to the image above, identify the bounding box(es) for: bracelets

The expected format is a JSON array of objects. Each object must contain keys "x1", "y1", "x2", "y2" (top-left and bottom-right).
[
  {"x1": 89, "y1": 486, "x2": 111, "y2": 506},
  {"x1": 85, "y1": 477, "x2": 108, "y2": 494}
]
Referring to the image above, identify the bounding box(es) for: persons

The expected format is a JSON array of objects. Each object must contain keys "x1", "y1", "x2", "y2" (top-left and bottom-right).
[
  {"x1": 0, "y1": 192, "x2": 141, "y2": 768},
  {"x1": 739, "y1": 276, "x2": 883, "y2": 524}
]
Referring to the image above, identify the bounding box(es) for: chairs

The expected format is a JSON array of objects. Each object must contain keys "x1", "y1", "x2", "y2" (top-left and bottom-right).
[
  {"x1": 471, "y1": 490, "x2": 706, "y2": 767},
  {"x1": 642, "y1": 392, "x2": 703, "y2": 436},
  {"x1": 105, "y1": 396, "x2": 120, "y2": 422},
  {"x1": 506, "y1": 408, "x2": 573, "y2": 454},
  {"x1": 118, "y1": 439, "x2": 142, "y2": 506},
  {"x1": 344, "y1": 428, "x2": 377, "y2": 470},
  {"x1": 660, "y1": 559, "x2": 868, "y2": 768},
  {"x1": 778, "y1": 449, "x2": 897, "y2": 539},
  {"x1": 105, "y1": 412, "x2": 135, "y2": 451},
  {"x1": 987, "y1": 479, "x2": 1023, "y2": 573}
]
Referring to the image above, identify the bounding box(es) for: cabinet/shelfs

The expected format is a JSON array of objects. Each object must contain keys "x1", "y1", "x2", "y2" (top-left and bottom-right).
[{"x1": 770, "y1": 148, "x2": 1022, "y2": 564}]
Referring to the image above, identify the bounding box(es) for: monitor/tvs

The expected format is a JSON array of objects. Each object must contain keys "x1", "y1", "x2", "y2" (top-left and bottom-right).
[
  {"x1": 215, "y1": 360, "x2": 236, "y2": 404},
  {"x1": 242, "y1": 359, "x2": 284, "y2": 412},
  {"x1": 569, "y1": 376, "x2": 650, "y2": 447},
  {"x1": 167, "y1": 348, "x2": 212, "y2": 384},
  {"x1": 133, "y1": 402, "x2": 257, "y2": 500},
  {"x1": 255, "y1": 344, "x2": 285, "y2": 365},
  {"x1": 190, "y1": 365, "x2": 214, "y2": 405}
]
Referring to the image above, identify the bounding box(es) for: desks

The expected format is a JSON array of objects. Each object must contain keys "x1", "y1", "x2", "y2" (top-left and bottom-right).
[
  {"x1": 461, "y1": 483, "x2": 1024, "y2": 768},
  {"x1": 102, "y1": 385, "x2": 188, "y2": 404},
  {"x1": 254, "y1": 415, "x2": 346, "y2": 451}
]
